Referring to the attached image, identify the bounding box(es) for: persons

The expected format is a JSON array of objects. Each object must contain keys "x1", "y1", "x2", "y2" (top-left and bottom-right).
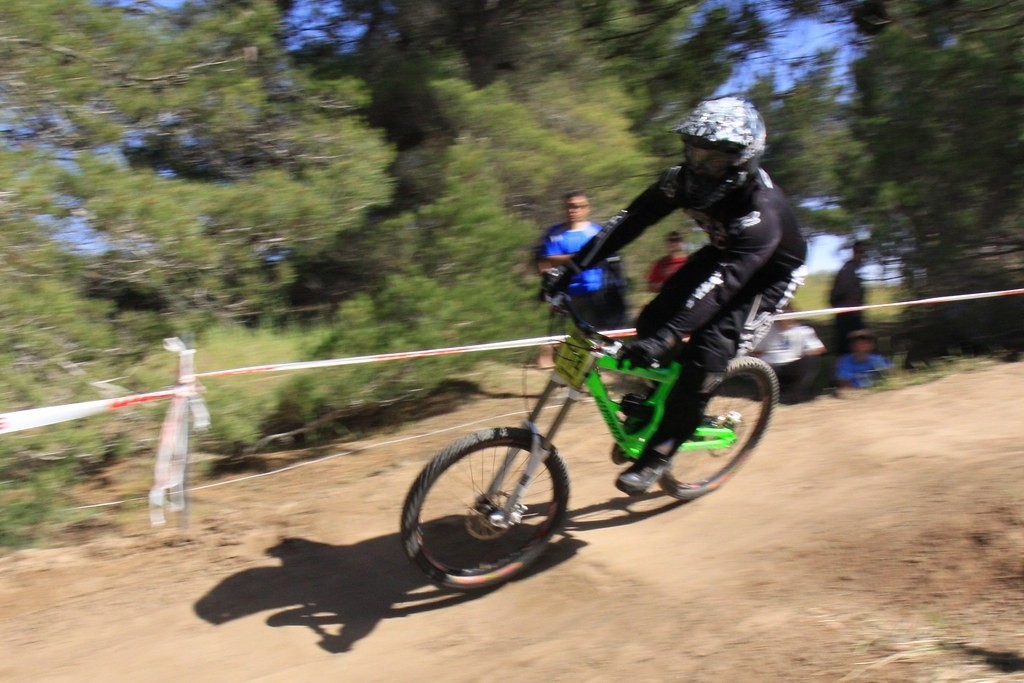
[
  {"x1": 747, "y1": 305, "x2": 828, "y2": 405},
  {"x1": 834, "y1": 328, "x2": 899, "y2": 392},
  {"x1": 542, "y1": 96, "x2": 811, "y2": 495},
  {"x1": 539, "y1": 191, "x2": 608, "y2": 329},
  {"x1": 825, "y1": 238, "x2": 875, "y2": 346},
  {"x1": 644, "y1": 232, "x2": 689, "y2": 294}
]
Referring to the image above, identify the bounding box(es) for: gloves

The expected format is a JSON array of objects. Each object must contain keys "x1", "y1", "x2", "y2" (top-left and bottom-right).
[
  {"x1": 540, "y1": 259, "x2": 579, "y2": 300},
  {"x1": 617, "y1": 336, "x2": 672, "y2": 371}
]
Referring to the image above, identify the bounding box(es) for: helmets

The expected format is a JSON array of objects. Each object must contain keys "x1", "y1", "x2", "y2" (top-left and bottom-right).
[{"x1": 669, "y1": 97, "x2": 765, "y2": 210}]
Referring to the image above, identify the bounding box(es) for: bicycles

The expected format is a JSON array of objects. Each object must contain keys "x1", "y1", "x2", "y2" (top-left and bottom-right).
[{"x1": 400, "y1": 270, "x2": 781, "y2": 593}]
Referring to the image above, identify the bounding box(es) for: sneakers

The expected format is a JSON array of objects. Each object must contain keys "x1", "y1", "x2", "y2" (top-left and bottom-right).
[{"x1": 614, "y1": 443, "x2": 674, "y2": 494}]
